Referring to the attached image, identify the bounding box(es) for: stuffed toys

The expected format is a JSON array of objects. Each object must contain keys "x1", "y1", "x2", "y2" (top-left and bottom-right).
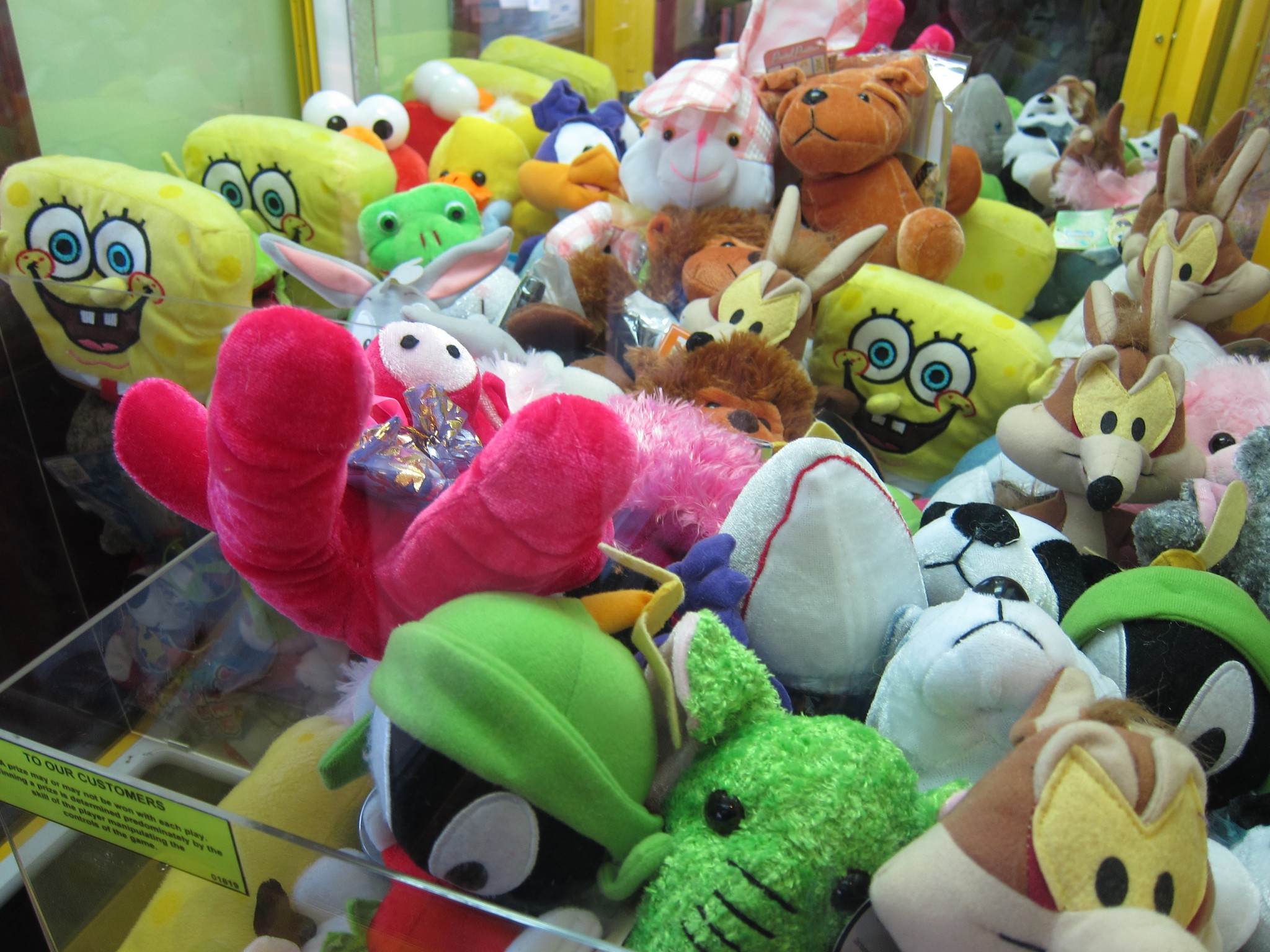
[
  {"x1": 0, "y1": 0, "x2": 1270, "y2": 951},
  {"x1": 115, "y1": 305, "x2": 640, "y2": 668}
]
